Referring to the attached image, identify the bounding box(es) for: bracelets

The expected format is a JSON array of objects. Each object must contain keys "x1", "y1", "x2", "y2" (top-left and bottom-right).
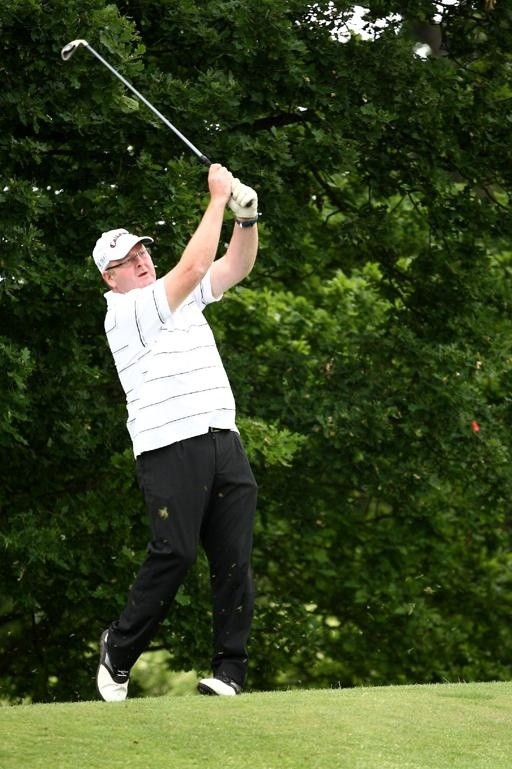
[{"x1": 235, "y1": 219, "x2": 258, "y2": 228}]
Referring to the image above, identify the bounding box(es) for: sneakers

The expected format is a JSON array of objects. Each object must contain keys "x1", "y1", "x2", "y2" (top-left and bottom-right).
[
  {"x1": 197, "y1": 678, "x2": 241, "y2": 696},
  {"x1": 97, "y1": 629, "x2": 129, "y2": 702}
]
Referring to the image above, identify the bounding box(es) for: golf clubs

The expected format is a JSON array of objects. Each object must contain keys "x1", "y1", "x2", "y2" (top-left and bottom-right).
[{"x1": 61, "y1": 37, "x2": 214, "y2": 166}]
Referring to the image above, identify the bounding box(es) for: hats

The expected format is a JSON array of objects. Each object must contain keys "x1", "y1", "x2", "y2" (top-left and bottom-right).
[{"x1": 93, "y1": 228, "x2": 155, "y2": 273}]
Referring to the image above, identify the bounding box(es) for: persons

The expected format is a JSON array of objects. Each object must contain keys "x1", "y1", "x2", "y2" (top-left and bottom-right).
[{"x1": 89, "y1": 159, "x2": 263, "y2": 702}]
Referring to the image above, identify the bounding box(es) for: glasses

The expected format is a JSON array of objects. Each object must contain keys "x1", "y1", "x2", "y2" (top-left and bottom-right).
[{"x1": 105, "y1": 247, "x2": 151, "y2": 270}]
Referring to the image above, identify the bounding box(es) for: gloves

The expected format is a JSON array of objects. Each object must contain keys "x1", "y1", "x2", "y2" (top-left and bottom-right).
[{"x1": 226, "y1": 178, "x2": 258, "y2": 219}]
